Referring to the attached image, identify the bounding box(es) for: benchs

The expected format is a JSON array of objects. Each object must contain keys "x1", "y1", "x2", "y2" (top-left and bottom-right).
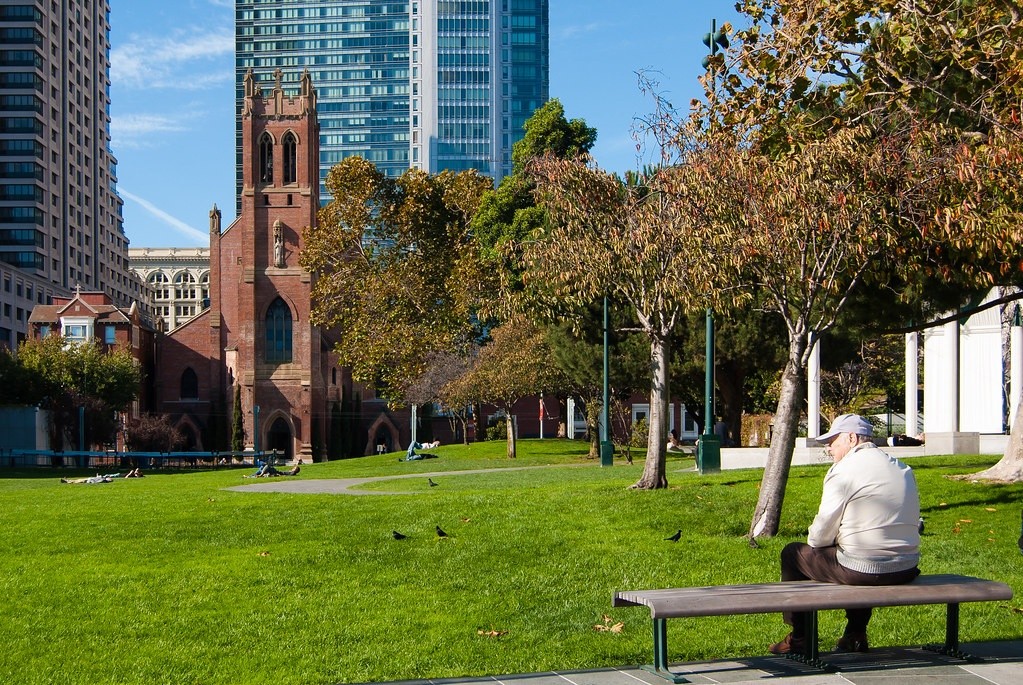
[{"x1": 612, "y1": 573, "x2": 1012, "y2": 684}]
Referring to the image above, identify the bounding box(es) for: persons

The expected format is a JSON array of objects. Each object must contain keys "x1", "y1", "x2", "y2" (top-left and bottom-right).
[
  {"x1": 97, "y1": 468, "x2": 144, "y2": 478},
  {"x1": 399, "y1": 440, "x2": 441, "y2": 462},
  {"x1": 768, "y1": 413, "x2": 919, "y2": 658},
  {"x1": 243, "y1": 464, "x2": 301, "y2": 479},
  {"x1": 60, "y1": 476, "x2": 112, "y2": 484}
]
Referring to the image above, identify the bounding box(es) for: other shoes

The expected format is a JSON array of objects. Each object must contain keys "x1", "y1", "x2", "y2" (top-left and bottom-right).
[
  {"x1": 836, "y1": 634, "x2": 868, "y2": 651},
  {"x1": 769, "y1": 633, "x2": 818, "y2": 654}
]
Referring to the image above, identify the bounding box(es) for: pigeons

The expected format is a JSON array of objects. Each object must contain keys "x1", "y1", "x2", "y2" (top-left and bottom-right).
[
  {"x1": 427, "y1": 478, "x2": 439, "y2": 487},
  {"x1": 436, "y1": 526, "x2": 448, "y2": 537},
  {"x1": 398, "y1": 459, "x2": 403, "y2": 462},
  {"x1": 918, "y1": 516, "x2": 925, "y2": 534},
  {"x1": 664, "y1": 529, "x2": 683, "y2": 542},
  {"x1": 392, "y1": 530, "x2": 407, "y2": 540}
]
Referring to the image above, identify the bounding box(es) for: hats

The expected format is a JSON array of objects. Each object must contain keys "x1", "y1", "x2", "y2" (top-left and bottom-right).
[{"x1": 815, "y1": 413, "x2": 873, "y2": 444}]
